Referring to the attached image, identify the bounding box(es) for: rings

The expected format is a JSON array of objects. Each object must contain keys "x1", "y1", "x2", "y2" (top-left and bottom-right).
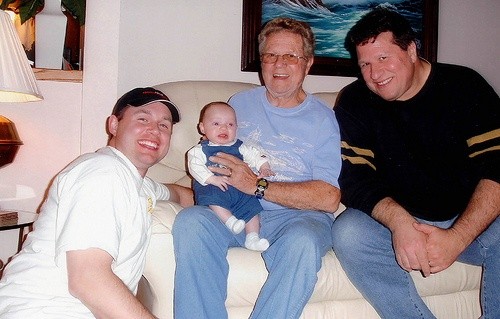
[{"x1": 228, "y1": 169, "x2": 232, "y2": 177}]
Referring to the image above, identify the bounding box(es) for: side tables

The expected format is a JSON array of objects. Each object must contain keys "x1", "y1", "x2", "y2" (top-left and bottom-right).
[{"x1": 0, "y1": 209, "x2": 40, "y2": 254}]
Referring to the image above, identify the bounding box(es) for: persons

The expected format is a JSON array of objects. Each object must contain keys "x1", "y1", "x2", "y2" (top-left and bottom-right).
[
  {"x1": 0, "y1": 88, "x2": 181, "y2": 319},
  {"x1": 171, "y1": 16, "x2": 342, "y2": 319},
  {"x1": 332, "y1": 6, "x2": 500, "y2": 319}
]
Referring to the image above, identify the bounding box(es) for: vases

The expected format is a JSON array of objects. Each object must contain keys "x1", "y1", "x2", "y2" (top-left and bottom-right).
[{"x1": 34, "y1": 0, "x2": 68, "y2": 70}]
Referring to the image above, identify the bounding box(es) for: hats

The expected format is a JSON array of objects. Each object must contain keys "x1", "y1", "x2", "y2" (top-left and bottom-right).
[{"x1": 112, "y1": 87, "x2": 181, "y2": 123}]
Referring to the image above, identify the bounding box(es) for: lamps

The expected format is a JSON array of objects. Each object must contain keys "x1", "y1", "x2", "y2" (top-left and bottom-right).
[{"x1": 0, "y1": 9, "x2": 44, "y2": 224}]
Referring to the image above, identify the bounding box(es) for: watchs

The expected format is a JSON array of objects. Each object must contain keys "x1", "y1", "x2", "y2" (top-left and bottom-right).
[{"x1": 255, "y1": 178, "x2": 270, "y2": 199}]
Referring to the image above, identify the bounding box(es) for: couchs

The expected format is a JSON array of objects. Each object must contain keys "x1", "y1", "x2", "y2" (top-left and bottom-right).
[{"x1": 136, "y1": 81, "x2": 483, "y2": 319}]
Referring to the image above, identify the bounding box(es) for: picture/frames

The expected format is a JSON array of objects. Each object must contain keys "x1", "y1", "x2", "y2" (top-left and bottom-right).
[{"x1": 241, "y1": 0, "x2": 439, "y2": 78}]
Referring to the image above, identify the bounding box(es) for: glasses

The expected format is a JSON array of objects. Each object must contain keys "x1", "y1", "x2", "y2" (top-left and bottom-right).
[{"x1": 260, "y1": 53, "x2": 308, "y2": 65}]
